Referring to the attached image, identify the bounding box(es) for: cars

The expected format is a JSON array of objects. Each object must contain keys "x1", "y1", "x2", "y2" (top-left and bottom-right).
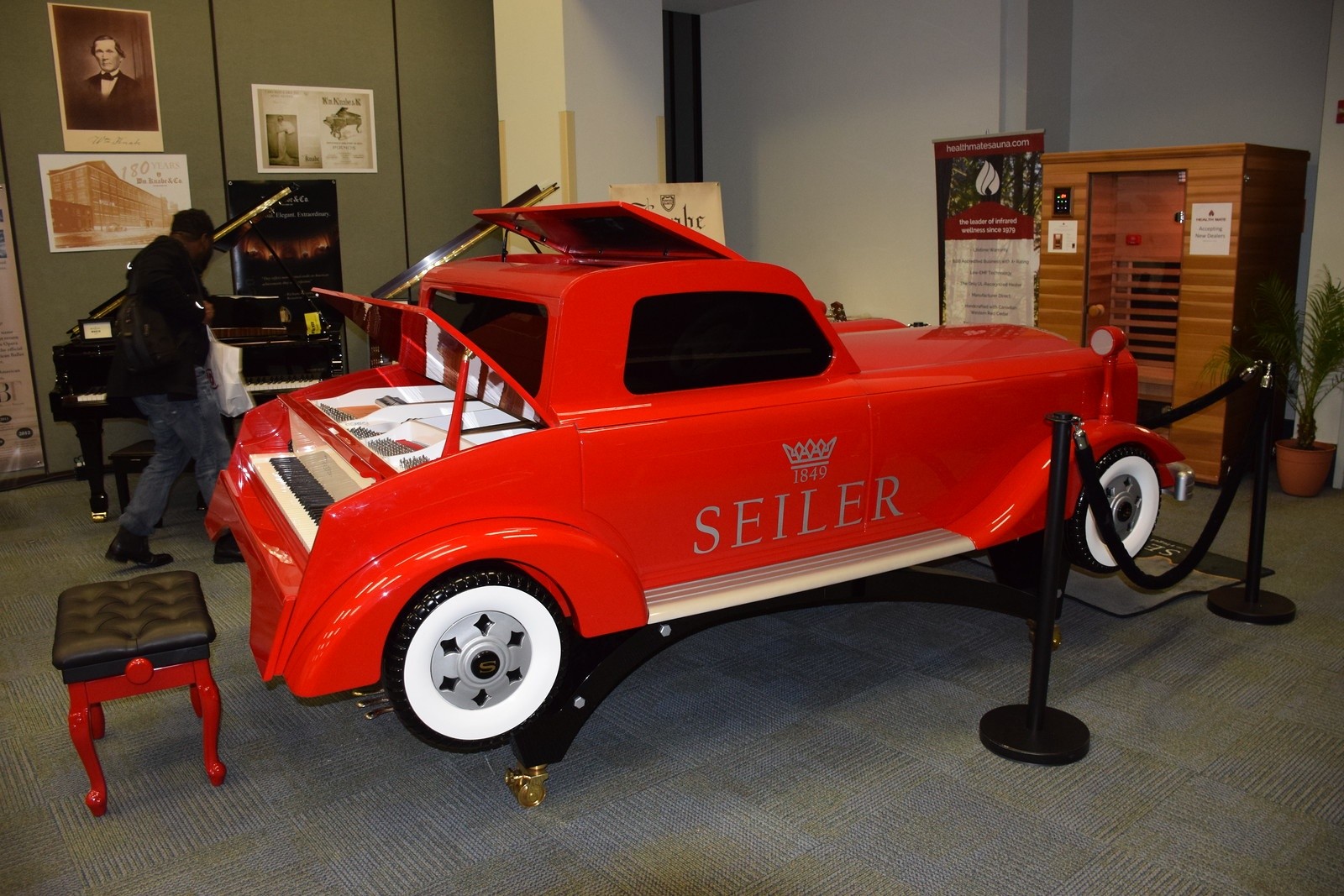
[{"x1": 201, "y1": 199, "x2": 1184, "y2": 757}]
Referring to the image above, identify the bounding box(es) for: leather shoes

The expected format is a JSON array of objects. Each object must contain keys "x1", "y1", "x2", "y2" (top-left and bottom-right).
[
  {"x1": 213, "y1": 533, "x2": 245, "y2": 564},
  {"x1": 105, "y1": 526, "x2": 173, "y2": 568}
]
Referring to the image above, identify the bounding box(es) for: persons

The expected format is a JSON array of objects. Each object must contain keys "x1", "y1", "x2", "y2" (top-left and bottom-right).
[
  {"x1": 105, "y1": 208, "x2": 247, "y2": 565},
  {"x1": 81, "y1": 34, "x2": 143, "y2": 131}
]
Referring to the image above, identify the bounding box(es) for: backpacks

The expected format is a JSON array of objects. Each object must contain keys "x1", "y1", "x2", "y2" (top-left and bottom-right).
[{"x1": 121, "y1": 244, "x2": 204, "y2": 371}]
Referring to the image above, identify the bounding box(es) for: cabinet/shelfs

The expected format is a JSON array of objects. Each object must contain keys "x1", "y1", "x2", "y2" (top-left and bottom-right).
[{"x1": 1038, "y1": 143, "x2": 1311, "y2": 486}]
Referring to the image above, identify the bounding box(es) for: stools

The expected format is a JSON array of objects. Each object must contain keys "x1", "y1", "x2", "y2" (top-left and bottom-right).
[
  {"x1": 52, "y1": 571, "x2": 228, "y2": 817},
  {"x1": 108, "y1": 439, "x2": 163, "y2": 529}
]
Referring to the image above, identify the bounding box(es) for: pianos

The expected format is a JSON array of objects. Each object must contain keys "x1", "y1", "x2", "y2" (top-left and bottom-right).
[
  {"x1": 249, "y1": 378, "x2": 559, "y2": 574},
  {"x1": 324, "y1": 107, "x2": 363, "y2": 140},
  {"x1": 367, "y1": 178, "x2": 559, "y2": 369},
  {"x1": 48, "y1": 187, "x2": 349, "y2": 522}
]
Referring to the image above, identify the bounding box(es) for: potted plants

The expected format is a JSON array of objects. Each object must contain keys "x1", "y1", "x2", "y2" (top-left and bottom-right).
[{"x1": 1194, "y1": 262, "x2": 1344, "y2": 496}]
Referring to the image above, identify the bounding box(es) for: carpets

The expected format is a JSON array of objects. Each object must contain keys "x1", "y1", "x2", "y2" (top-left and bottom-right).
[{"x1": 960, "y1": 535, "x2": 1275, "y2": 619}]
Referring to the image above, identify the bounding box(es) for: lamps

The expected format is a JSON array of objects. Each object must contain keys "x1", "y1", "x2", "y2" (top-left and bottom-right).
[{"x1": 72, "y1": 455, "x2": 86, "y2": 481}]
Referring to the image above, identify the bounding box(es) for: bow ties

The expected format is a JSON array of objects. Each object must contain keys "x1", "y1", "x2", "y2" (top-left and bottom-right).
[{"x1": 102, "y1": 72, "x2": 117, "y2": 80}]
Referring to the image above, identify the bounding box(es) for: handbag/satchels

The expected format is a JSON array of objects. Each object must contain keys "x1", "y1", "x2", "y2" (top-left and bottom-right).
[{"x1": 205, "y1": 324, "x2": 255, "y2": 418}]
